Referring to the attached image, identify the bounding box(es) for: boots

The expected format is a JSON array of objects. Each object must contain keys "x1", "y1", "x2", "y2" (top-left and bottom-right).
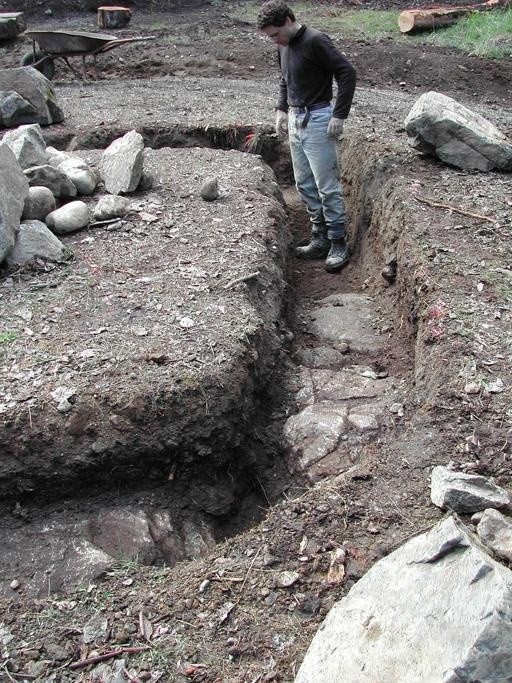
[
  {"x1": 295, "y1": 232, "x2": 330, "y2": 260},
  {"x1": 324, "y1": 238, "x2": 352, "y2": 273}
]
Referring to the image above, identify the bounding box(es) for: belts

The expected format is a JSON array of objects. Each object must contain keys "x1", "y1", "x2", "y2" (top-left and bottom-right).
[{"x1": 288, "y1": 100, "x2": 330, "y2": 128}]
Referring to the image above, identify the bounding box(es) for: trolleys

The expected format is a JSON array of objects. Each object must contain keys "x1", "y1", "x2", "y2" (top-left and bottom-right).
[{"x1": 19, "y1": 28, "x2": 156, "y2": 86}]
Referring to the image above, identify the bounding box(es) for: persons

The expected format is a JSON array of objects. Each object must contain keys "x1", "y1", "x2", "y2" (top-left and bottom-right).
[{"x1": 256, "y1": 0, "x2": 358, "y2": 273}]
